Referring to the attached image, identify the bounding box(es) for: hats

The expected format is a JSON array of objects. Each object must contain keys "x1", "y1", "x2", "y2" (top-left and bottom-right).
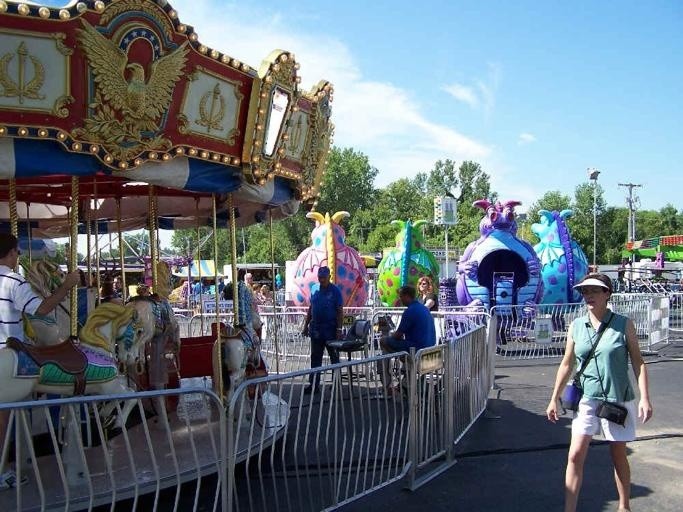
[
  {"x1": 318, "y1": 267, "x2": 329, "y2": 277},
  {"x1": 572, "y1": 278, "x2": 610, "y2": 289}
]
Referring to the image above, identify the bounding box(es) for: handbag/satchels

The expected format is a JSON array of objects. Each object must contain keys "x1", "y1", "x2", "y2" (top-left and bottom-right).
[
  {"x1": 562, "y1": 380, "x2": 580, "y2": 410},
  {"x1": 595, "y1": 401, "x2": 629, "y2": 424}
]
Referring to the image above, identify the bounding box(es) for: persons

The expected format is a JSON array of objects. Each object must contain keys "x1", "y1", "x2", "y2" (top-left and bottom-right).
[
  {"x1": 545, "y1": 273, "x2": 653, "y2": 511},
  {"x1": 373, "y1": 285, "x2": 436, "y2": 400},
  {"x1": 302, "y1": 266, "x2": 346, "y2": 395},
  {"x1": 416, "y1": 276, "x2": 439, "y2": 311},
  {"x1": 96, "y1": 269, "x2": 273, "y2": 346},
  {"x1": 0, "y1": 234, "x2": 81, "y2": 492}
]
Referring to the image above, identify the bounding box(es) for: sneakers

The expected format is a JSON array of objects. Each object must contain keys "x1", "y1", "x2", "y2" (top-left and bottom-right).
[
  {"x1": 304, "y1": 385, "x2": 318, "y2": 394},
  {"x1": 0, "y1": 470, "x2": 30, "y2": 492}
]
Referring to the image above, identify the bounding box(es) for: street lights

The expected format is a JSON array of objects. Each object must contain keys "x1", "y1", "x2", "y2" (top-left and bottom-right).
[{"x1": 589, "y1": 169, "x2": 600, "y2": 272}]
